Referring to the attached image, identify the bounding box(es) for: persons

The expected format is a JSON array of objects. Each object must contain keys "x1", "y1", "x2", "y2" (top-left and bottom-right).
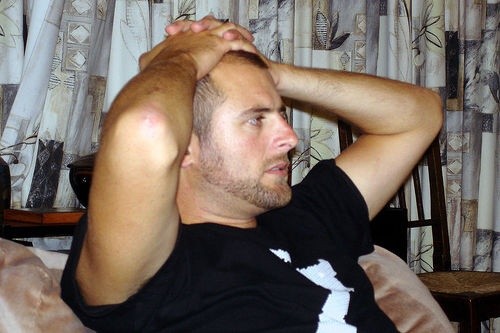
[{"x1": 60, "y1": 15, "x2": 444, "y2": 333}]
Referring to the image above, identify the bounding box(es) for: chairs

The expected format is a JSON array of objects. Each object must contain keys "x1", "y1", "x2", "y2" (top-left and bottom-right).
[{"x1": 338, "y1": 120, "x2": 500, "y2": 333}]
